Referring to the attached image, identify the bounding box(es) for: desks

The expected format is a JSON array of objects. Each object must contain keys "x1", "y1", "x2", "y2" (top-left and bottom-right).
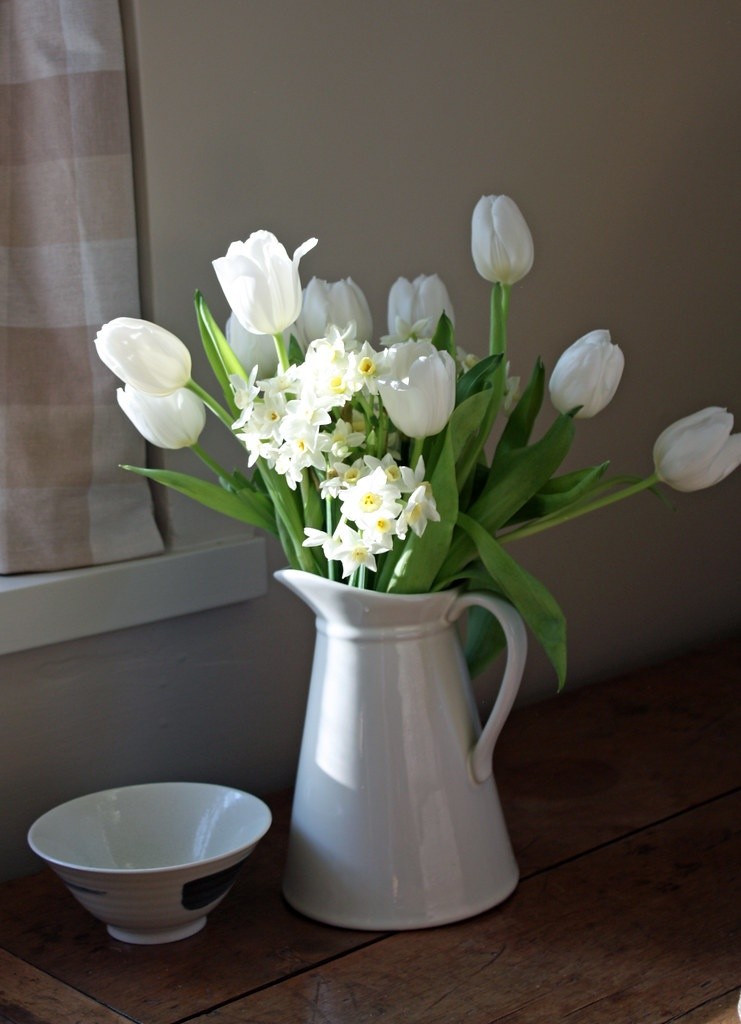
[{"x1": 0, "y1": 621, "x2": 740, "y2": 1024}]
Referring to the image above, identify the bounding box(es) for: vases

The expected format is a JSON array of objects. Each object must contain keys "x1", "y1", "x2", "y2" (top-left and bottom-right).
[{"x1": 273, "y1": 566, "x2": 528, "y2": 931}]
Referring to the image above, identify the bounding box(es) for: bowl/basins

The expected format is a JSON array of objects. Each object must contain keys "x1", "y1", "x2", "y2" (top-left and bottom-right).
[{"x1": 27, "y1": 781, "x2": 273, "y2": 945}]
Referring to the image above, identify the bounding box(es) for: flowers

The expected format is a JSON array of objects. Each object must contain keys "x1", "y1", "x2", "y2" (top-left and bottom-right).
[{"x1": 93, "y1": 195, "x2": 741, "y2": 695}]
